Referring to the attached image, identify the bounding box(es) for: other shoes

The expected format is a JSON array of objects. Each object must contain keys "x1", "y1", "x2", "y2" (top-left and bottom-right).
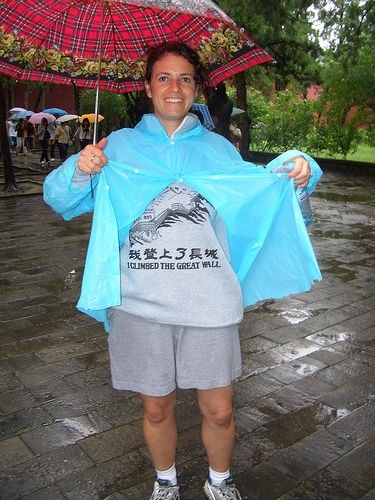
[
  {"x1": 51, "y1": 158, "x2": 55, "y2": 162},
  {"x1": 42, "y1": 161, "x2": 45, "y2": 164}
]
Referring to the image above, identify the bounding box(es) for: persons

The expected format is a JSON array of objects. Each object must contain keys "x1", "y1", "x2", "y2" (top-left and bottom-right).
[
  {"x1": 229, "y1": 117, "x2": 242, "y2": 153},
  {"x1": 42, "y1": 41, "x2": 323, "y2": 500},
  {"x1": 6, "y1": 116, "x2": 36, "y2": 156},
  {"x1": 55, "y1": 121, "x2": 69, "y2": 161},
  {"x1": 47, "y1": 113, "x2": 60, "y2": 161},
  {"x1": 73, "y1": 118, "x2": 93, "y2": 149},
  {"x1": 38, "y1": 117, "x2": 51, "y2": 166}
]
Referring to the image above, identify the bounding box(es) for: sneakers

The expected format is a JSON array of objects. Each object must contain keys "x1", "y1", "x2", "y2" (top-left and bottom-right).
[
  {"x1": 150, "y1": 479, "x2": 180, "y2": 500},
  {"x1": 203, "y1": 477, "x2": 243, "y2": 500}
]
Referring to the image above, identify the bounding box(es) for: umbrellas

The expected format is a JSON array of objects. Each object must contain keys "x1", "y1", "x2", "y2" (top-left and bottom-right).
[
  {"x1": 7, "y1": 108, "x2": 104, "y2": 124},
  {"x1": 0, "y1": 0, "x2": 275, "y2": 174},
  {"x1": 191, "y1": 103, "x2": 246, "y2": 130}
]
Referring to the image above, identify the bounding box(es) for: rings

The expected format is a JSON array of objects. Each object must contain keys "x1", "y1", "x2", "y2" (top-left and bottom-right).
[
  {"x1": 91, "y1": 154, "x2": 96, "y2": 162},
  {"x1": 308, "y1": 172, "x2": 312, "y2": 178}
]
